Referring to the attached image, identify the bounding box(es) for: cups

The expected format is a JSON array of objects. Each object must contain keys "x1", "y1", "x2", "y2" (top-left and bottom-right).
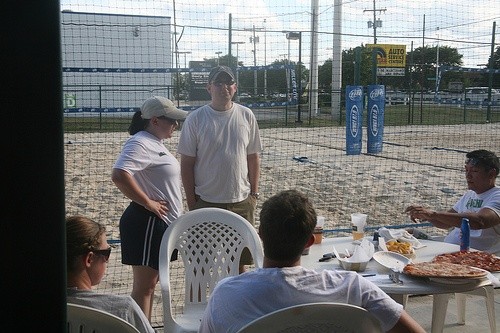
[
  {"x1": 313, "y1": 215, "x2": 325, "y2": 244},
  {"x1": 351, "y1": 213, "x2": 368, "y2": 240}
]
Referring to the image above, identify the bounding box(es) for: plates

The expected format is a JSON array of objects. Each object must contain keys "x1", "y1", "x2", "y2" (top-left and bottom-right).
[
  {"x1": 426, "y1": 267, "x2": 491, "y2": 284},
  {"x1": 372, "y1": 250, "x2": 414, "y2": 271}
]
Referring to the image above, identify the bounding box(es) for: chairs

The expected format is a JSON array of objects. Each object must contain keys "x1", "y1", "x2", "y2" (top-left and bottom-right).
[
  {"x1": 67, "y1": 303, "x2": 141, "y2": 333},
  {"x1": 159, "y1": 208, "x2": 265, "y2": 333},
  {"x1": 238, "y1": 302, "x2": 387, "y2": 333},
  {"x1": 430, "y1": 291, "x2": 496, "y2": 333}
]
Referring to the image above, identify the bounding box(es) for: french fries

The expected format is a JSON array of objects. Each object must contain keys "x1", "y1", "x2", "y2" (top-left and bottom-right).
[{"x1": 386, "y1": 240, "x2": 412, "y2": 255}]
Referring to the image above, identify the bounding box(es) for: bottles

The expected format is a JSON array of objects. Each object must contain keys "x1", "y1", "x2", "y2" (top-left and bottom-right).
[{"x1": 460, "y1": 218, "x2": 471, "y2": 251}]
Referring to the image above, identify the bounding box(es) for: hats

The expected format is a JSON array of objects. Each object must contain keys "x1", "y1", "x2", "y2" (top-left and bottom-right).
[
  {"x1": 141, "y1": 95, "x2": 188, "y2": 120},
  {"x1": 208, "y1": 66, "x2": 234, "y2": 80}
]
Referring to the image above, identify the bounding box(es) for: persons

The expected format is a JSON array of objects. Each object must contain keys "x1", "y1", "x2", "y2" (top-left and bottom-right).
[
  {"x1": 405, "y1": 149, "x2": 500, "y2": 257},
  {"x1": 177, "y1": 66, "x2": 263, "y2": 272},
  {"x1": 199, "y1": 189, "x2": 427, "y2": 333},
  {"x1": 111, "y1": 95, "x2": 187, "y2": 324},
  {"x1": 65, "y1": 217, "x2": 156, "y2": 333}
]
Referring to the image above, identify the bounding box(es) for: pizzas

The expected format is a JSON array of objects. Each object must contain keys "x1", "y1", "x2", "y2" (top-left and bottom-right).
[
  {"x1": 404, "y1": 262, "x2": 488, "y2": 278},
  {"x1": 434, "y1": 250, "x2": 500, "y2": 271}
]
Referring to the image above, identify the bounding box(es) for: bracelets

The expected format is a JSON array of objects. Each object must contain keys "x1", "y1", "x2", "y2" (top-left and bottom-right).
[{"x1": 251, "y1": 192, "x2": 259, "y2": 199}]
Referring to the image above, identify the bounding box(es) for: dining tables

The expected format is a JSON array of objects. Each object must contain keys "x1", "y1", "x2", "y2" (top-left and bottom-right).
[{"x1": 301, "y1": 235, "x2": 500, "y2": 333}]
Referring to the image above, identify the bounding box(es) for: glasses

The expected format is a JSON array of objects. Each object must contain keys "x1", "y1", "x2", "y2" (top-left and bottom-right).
[
  {"x1": 210, "y1": 79, "x2": 235, "y2": 87},
  {"x1": 158, "y1": 116, "x2": 177, "y2": 125},
  {"x1": 82, "y1": 248, "x2": 111, "y2": 261},
  {"x1": 464, "y1": 157, "x2": 491, "y2": 170}
]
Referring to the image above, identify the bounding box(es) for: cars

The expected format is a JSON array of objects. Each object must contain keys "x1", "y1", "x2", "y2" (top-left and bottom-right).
[{"x1": 461, "y1": 86, "x2": 500, "y2": 103}]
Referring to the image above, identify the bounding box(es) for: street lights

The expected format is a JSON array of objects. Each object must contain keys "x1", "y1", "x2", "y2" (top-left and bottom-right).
[
  {"x1": 286, "y1": 32, "x2": 300, "y2": 65},
  {"x1": 231, "y1": 42, "x2": 244, "y2": 95},
  {"x1": 180, "y1": 52, "x2": 191, "y2": 82},
  {"x1": 216, "y1": 52, "x2": 223, "y2": 66}
]
